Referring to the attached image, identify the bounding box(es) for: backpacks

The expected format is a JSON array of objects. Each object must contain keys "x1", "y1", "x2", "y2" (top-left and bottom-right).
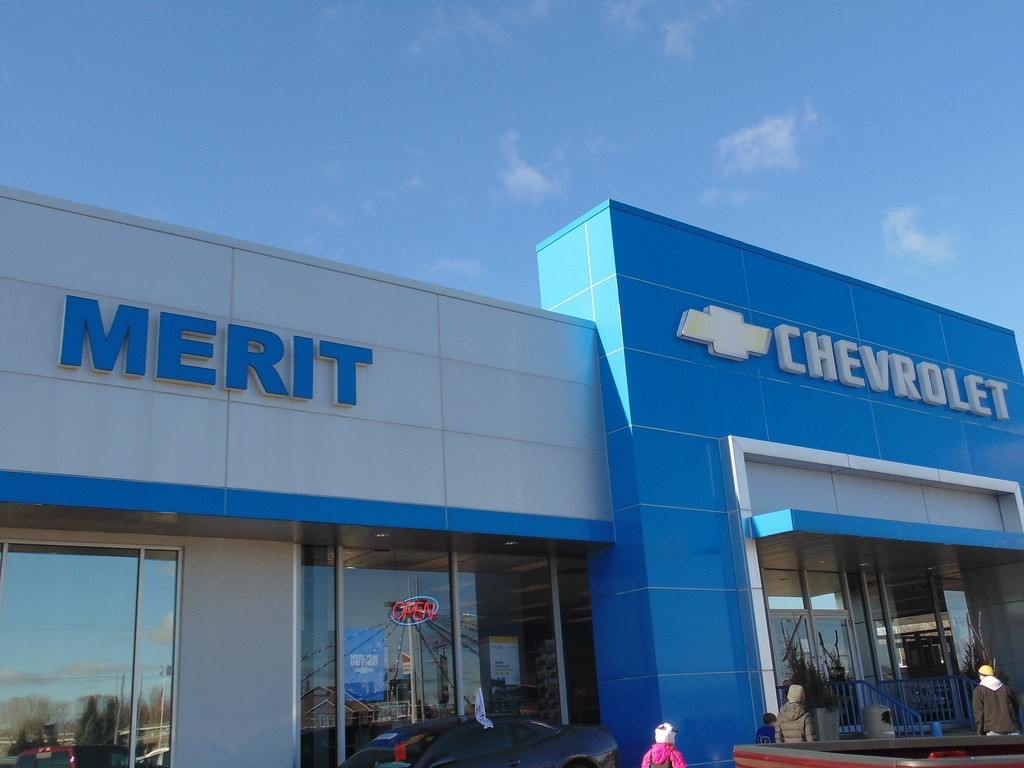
[{"x1": 649, "y1": 750, "x2": 672, "y2": 768}]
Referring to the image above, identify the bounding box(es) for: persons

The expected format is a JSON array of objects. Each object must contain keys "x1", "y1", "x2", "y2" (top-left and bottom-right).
[
  {"x1": 641, "y1": 723, "x2": 687, "y2": 768},
  {"x1": 755, "y1": 713, "x2": 777, "y2": 744},
  {"x1": 773, "y1": 685, "x2": 818, "y2": 743},
  {"x1": 973, "y1": 664, "x2": 1022, "y2": 736}
]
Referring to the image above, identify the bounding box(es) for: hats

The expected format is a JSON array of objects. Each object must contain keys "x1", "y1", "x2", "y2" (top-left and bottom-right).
[
  {"x1": 978, "y1": 665, "x2": 994, "y2": 675},
  {"x1": 763, "y1": 713, "x2": 776, "y2": 725},
  {"x1": 655, "y1": 722, "x2": 678, "y2": 743},
  {"x1": 787, "y1": 684, "x2": 805, "y2": 702}
]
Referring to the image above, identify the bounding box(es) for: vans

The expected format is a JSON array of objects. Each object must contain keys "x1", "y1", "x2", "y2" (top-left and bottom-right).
[{"x1": 15, "y1": 745, "x2": 145, "y2": 768}]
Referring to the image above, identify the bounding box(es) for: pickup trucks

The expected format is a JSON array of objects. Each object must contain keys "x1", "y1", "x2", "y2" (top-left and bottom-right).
[{"x1": 736, "y1": 735, "x2": 1024, "y2": 768}]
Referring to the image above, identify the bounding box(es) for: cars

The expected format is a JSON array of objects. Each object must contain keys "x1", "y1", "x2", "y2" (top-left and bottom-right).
[
  {"x1": 881, "y1": 665, "x2": 952, "y2": 709},
  {"x1": 336, "y1": 711, "x2": 618, "y2": 768}
]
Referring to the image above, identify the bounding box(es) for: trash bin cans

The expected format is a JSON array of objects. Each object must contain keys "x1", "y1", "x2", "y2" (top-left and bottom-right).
[{"x1": 862, "y1": 704, "x2": 895, "y2": 737}]
